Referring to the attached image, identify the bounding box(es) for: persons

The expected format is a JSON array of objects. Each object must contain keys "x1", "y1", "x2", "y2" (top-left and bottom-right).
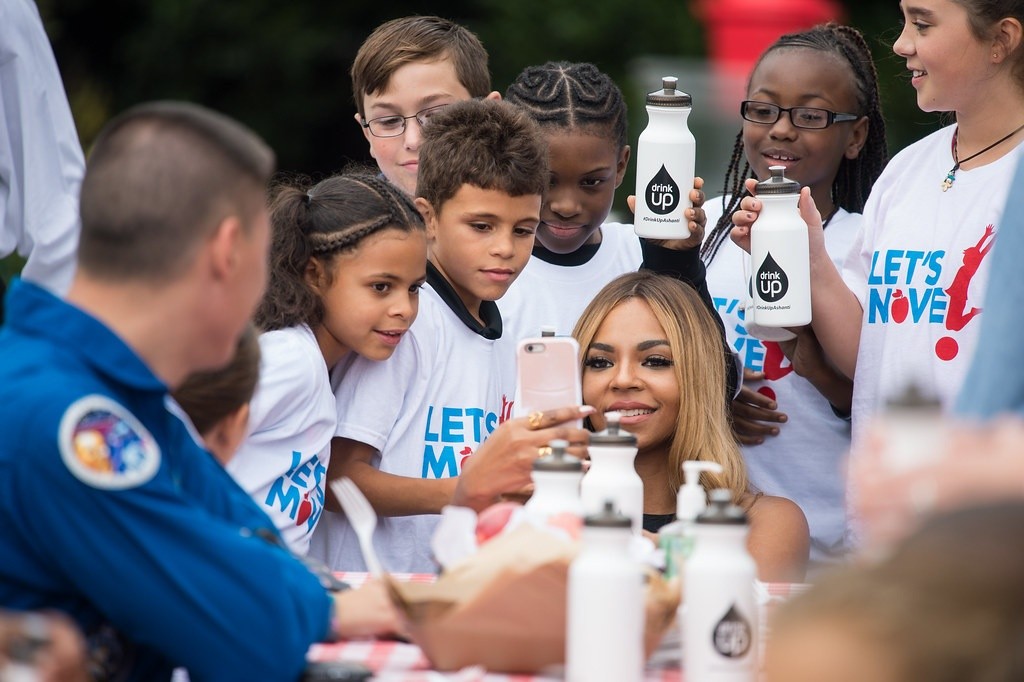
[{"x1": 1, "y1": 0, "x2": 1024, "y2": 682}]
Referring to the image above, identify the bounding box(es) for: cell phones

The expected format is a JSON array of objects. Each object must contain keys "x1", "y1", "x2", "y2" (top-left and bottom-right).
[{"x1": 517, "y1": 337, "x2": 583, "y2": 430}]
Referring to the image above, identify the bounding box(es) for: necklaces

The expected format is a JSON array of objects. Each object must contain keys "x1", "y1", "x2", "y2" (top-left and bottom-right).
[{"x1": 943, "y1": 124, "x2": 1023, "y2": 192}]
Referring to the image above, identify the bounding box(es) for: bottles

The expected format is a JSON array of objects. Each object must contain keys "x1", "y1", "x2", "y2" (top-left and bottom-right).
[
  {"x1": 633, "y1": 77, "x2": 696, "y2": 240},
  {"x1": 745, "y1": 165, "x2": 812, "y2": 339},
  {"x1": 679, "y1": 486, "x2": 758, "y2": 682},
  {"x1": 880, "y1": 382, "x2": 945, "y2": 474},
  {"x1": 474, "y1": 412, "x2": 646, "y2": 682}
]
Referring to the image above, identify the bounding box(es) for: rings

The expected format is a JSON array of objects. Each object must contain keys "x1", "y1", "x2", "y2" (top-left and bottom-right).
[
  {"x1": 538, "y1": 446, "x2": 553, "y2": 456},
  {"x1": 527, "y1": 410, "x2": 546, "y2": 429}
]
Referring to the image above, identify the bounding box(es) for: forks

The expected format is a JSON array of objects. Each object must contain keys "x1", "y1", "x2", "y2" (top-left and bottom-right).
[{"x1": 328, "y1": 475, "x2": 386, "y2": 582}]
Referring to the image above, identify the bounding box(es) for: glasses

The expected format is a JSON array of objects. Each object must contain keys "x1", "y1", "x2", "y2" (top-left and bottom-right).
[
  {"x1": 741, "y1": 101, "x2": 862, "y2": 129},
  {"x1": 361, "y1": 103, "x2": 449, "y2": 138}
]
